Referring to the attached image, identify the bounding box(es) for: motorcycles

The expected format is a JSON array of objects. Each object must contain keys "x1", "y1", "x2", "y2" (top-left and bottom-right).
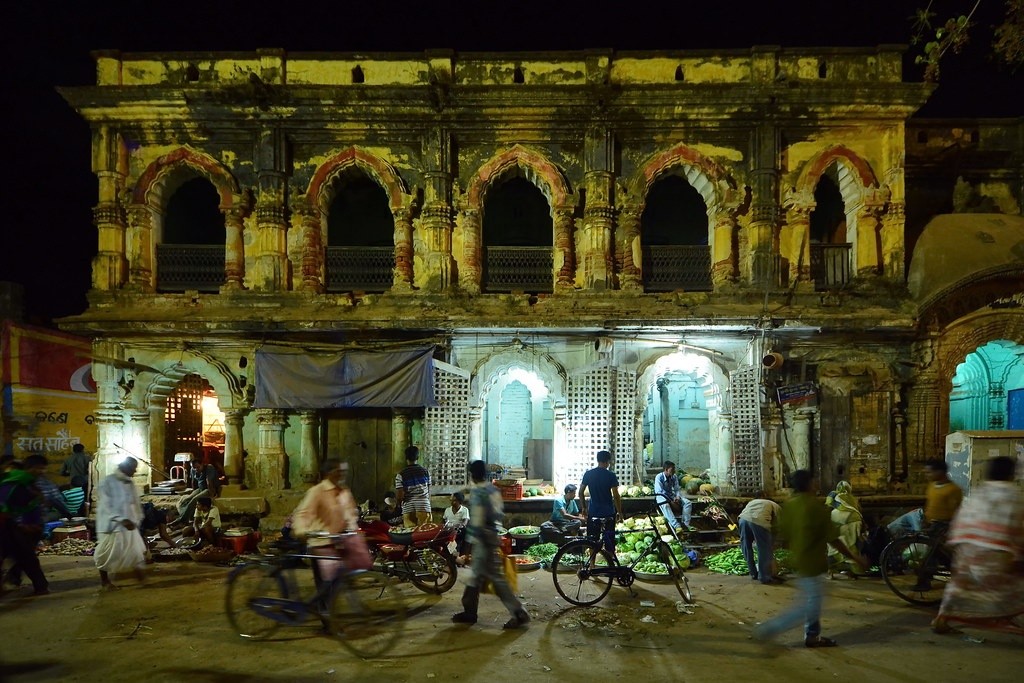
[{"x1": 352, "y1": 501, "x2": 465, "y2": 595}]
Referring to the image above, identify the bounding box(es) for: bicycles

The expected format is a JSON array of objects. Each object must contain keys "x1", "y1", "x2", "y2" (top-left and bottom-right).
[
  {"x1": 879, "y1": 517, "x2": 958, "y2": 607},
  {"x1": 220, "y1": 530, "x2": 408, "y2": 660},
  {"x1": 552, "y1": 498, "x2": 692, "y2": 605}
]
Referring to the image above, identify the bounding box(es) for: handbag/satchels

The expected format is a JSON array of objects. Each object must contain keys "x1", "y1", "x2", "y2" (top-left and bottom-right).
[
  {"x1": 888, "y1": 508, "x2": 928, "y2": 560},
  {"x1": 481, "y1": 550, "x2": 517, "y2": 594}
]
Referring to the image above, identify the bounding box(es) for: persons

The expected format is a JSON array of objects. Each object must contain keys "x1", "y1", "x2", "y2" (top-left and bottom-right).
[
  {"x1": 909, "y1": 458, "x2": 962, "y2": 592},
  {"x1": 442, "y1": 493, "x2": 470, "y2": 564},
  {"x1": 540, "y1": 485, "x2": 587, "y2": 546},
  {"x1": 94, "y1": 457, "x2": 149, "y2": 592},
  {"x1": 579, "y1": 451, "x2": 623, "y2": 582},
  {"x1": 655, "y1": 460, "x2": 698, "y2": 535},
  {"x1": 193, "y1": 498, "x2": 222, "y2": 545},
  {"x1": 738, "y1": 499, "x2": 784, "y2": 585},
  {"x1": 453, "y1": 461, "x2": 530, "y2": 630},
  {"x1": 0, "y1": 454, "x2": 79, "y2": 595},
  {"x1": 166, "y1": 459, "x2": 222, "y2": 528},
  {"x1": 62, "y1": 444, "x2": 92, "y2": 500},
  {"x1": 929, "y1": 457, "x2": 1024, "y2": 634},
  {"x1": 291, "y1": 457, "x2": 372, "y2": 636},
  {"x1": 139, "y1": 502, "x2": 179, "y2": 554},
  {"x1": 750, "y1": 470, "x2": 838, "y2": 648},
  {"x1": 380, "y1": 446, "x2": 432, "y2": 528}
]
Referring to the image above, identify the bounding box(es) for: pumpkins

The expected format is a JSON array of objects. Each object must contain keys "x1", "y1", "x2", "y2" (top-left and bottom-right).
[{"x1": 678, "y1": 473, "x2": 713, "y2": 495}]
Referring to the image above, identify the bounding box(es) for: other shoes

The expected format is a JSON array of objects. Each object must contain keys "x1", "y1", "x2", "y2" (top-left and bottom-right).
[
  {"x1": 807, "y1": 637, "x2": 836, "y2": 647},
  {"x1": 931, "y1": 622, "x2": 963, "y2": 634},
  {"x1": 683, "y1": 526, "x2": 698, "y2": 531},
  {"x1": 101, "y1": 583, "x2": 121, "y2": 591},
  {"x1": 2, "y1": 582, "x2": 27, "y2": 591},
  {"x1": 676, "y1": 527, "x2": 684, "y2": 535},
  {"x1": 454, "y1": 612, "x2": 478, "y2": 622},
  {"x1": 42, "y1": 587, "x2": 55, "y2": 594},
  {"x1": 911, "y1": 583, "x2": 931, "y2": 591},
  {"x1": 504, "y1": 617, "x2": 530, "y2": 628},
  {"x1": 752, "y1": 573, "x2": 758, "y2": 579},
  {"x1": 135, "y1": 577, "x2": 161, "y2": 589}
]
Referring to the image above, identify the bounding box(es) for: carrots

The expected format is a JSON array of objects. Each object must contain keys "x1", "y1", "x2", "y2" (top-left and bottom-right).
[{"x1": 516, "y1": 559, "x2": 529, "y2": 564}]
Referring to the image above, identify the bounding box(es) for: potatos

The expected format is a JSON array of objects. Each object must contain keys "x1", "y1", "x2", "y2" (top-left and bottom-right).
[
  {"x1": 38, "y1": 537, "x2": 96, "y2": 555},
  {"x1": 196, "y1": 544, "x2": 227, "y2": 555}
]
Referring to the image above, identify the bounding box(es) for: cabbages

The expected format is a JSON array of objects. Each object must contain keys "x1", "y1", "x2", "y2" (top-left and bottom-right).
[
  {"x1": 614, "y1": 517, "x2": 690, "y2": 569},
  {"x1": 617, "y1": 485, "x2": 651, "y2": 496}
]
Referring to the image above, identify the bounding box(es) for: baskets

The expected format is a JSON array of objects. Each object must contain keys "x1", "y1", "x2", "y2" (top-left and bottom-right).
[
  {"x1": 511, "y1": 554, "x2": 543, "y2": 570},
  {"x1": 509, "y1": 526, "x2": 541, "y2": 539},
  {"x1": 159, "y1": 553, "x2": 189, "y2": 562},
  {"x1": 547, "y1": 560, "x2": 580, "y2": 571},
  {"x1": 632, "y1": 562, "x2": 669, "y2": 579},
  {"x1": 189, "y1": 550, "x2": 233, "y2": 562}
]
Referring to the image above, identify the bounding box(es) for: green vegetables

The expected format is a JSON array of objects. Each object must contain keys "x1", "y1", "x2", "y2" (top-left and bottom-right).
[
  {"x1": 510, "y1": 528, "x2": 539, "y2": 534},
  {"x1": 522, "y1": 543, "x2": 582, "y2": 564}
]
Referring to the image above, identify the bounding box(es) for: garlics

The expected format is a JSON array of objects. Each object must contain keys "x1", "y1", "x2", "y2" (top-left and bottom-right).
[{"x1": 159, "y1": 548, "x2": 189, "y2": 555}]
